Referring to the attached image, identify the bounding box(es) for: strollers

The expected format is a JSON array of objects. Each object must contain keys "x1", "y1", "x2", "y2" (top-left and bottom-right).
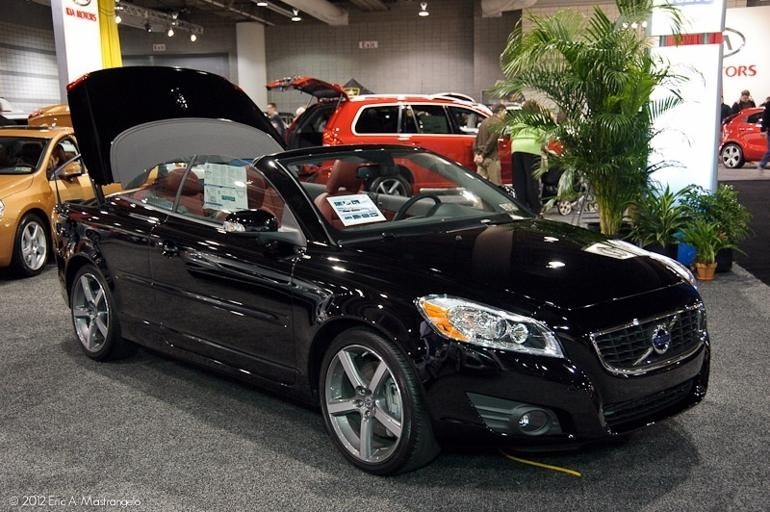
[{"x1": 536, "y1": 148, "x2": 601, "y2": 215}]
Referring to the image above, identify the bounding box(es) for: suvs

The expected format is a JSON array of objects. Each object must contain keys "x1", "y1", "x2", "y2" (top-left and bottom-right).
[{"x1": 266, "y1": 75, "x2": 577, "y2": 213}]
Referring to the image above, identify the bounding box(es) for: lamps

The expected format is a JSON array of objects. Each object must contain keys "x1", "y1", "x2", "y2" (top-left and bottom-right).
[
  {"x1": 417, "y1": 0, "x2": 430, "y2": 18},
  {"x1": 255, "y1": 0, "x2": 303, "y2": 22},
  {"x1": 112, "y1": 2, "x2": 208, "y2": 41}
]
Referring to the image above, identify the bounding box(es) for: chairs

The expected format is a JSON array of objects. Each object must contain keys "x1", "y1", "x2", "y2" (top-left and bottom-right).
[
  {"x1": 159, "y1": 166, "x2": 208, "y2": 216},
  {"x1": 212, "y1": 164, "x2": 276, "y2": 220},
  {"x1": 314, "y1": 158, "x2": 384, "y2": 231},
  {"x1": 21, "y1": 142, "x2": 42, "y2": 166},
  {"x1": 45, "y1": 144, "x2": 66, "y2": 166}
]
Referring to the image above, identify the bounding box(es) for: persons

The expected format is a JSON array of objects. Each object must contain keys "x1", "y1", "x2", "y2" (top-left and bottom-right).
[
  {"x1": 47, "y1": 144, "x2": 66, "y2": 179},
  {"x1": 266, "y1": 102, "x2": 284, "y2": 139},
  {"x1": 0, "y1": 144, "x2": 23, "y2": 165},
  {"x1": 452, "y1": 99, "x2": 576, "y2": 216},
  {"x1": 285, "y1": 107, "x2": 305, "y2": 148},
  {"x1": 721, "y1": 90, "x2": 770, "y2": 170}
]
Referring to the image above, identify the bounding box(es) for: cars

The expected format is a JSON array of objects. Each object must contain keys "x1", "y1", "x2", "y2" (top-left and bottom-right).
[
  {"x1": 1, "y1": 104, "x2": 159, "y2": 277},
  {"x1": 716, "y1": 106, "x2": 769, "y2": 171},
  {"x1": 49, "y1": 65, "x2": 712, "y2": 478}
]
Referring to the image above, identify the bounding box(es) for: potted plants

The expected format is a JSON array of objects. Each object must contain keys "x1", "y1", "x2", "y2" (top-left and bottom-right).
[
  {"x1": 692, "y1": 180, "x2": 750, "y2": 274},
  {"x1": 641, "y1": 184, "x2": 698, "y2": 258},
  {"x1": 486, "y1": 1, "x2": 708, "y2": 241},
  {"x1": 674, "y1": 217, "x2": 731, "y2": 285}
]
